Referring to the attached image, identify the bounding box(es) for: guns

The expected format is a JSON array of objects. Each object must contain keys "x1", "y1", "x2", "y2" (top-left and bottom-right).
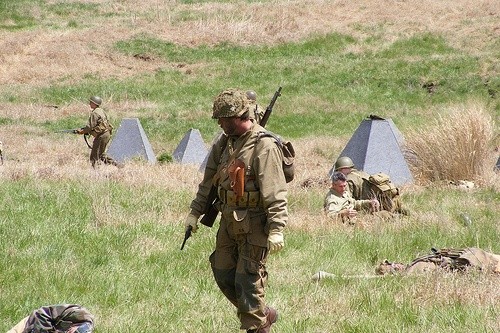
[
  {"x1": 180, "y1": 225, "x2": 192, "y2": 251},
  {"x1": 197, "y1": 86, "x2": 283, "y2": 227},
  {"x1": 54, "y1": 129, "x2": 93, "y2": 134}
]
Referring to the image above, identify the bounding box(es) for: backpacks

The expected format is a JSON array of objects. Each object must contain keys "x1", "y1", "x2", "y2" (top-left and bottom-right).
[
  {"x1": 254, "y1": 132, "x2": 296, "y2": 183},
  {"x1": 361, "y1": 173, "x2": 400, "y2": 214}
]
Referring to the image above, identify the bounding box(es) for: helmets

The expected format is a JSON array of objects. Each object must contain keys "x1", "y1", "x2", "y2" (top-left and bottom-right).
[
  {"x1": 334, "y1": 157, "x2": 354, "y2": 171},
  {"x1": 211, "y1": 89, "x2": 254, "y2": 120},
  {"x1": 89, "y1": 95, "x2": 102, "y2": 106},
  {"x1": 246, "y1": 91, "x2": 256, "y2": 101}
]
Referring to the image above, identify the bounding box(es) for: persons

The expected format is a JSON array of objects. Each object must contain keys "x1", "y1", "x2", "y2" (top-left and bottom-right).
[
  {"x1": 324, "y1": 172, "x2": 380, "y2": 218},
  {"x1": 185, "y1": 88, "x2": 288, "y2": 333},
  {"x1": 75, "y1": 96, "x2": 117, "y2": 166},
  {"x1": 377, "y1": 246, "x2": 500, "y2": 275},
  {"x1": 243, "y1": 90, "x2": 268, "y2": 129},
  {"x1": 6, "y1": 304, "x2": 94, "y2": 333},
  {"x1": 334, "y1": 156, "x2": 368, "y2": 200}
]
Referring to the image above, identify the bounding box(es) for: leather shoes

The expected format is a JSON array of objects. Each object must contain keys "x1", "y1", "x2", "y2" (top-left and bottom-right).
[{"x1": 248, "y1": 306, "x2": 277, "y2": 333}]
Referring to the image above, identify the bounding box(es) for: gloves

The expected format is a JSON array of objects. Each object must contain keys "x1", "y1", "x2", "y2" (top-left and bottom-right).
[
  {"x1": 267, "y1": 229, "x2": 284, "y2": 255},
  {"x1": 184, "y1": 213, "x2": 199, "y2": 233}
]
